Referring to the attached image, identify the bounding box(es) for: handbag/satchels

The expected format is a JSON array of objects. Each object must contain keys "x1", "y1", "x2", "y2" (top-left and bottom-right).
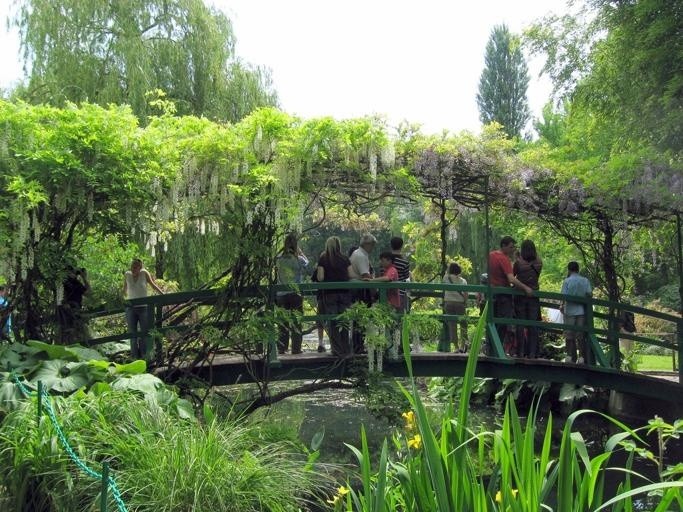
[{"x1": 317, "y1": 266, "x2": 325, "y2": 281}]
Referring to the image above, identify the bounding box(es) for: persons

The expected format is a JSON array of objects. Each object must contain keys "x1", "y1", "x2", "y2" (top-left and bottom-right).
[
  {"x1": 120, "y1": 259, "x2": 165, "y2": 361},
  {"x1": 60, "y1": 264, "x2": 93, "y2": 343},
  {"x1": 0, "y1": 283, "x2": 11, "y2": 344}
]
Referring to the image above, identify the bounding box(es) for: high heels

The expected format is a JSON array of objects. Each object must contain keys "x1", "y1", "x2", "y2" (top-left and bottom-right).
[{"x1": 318, "y1": 346, "x2": 326, "y2": 352}]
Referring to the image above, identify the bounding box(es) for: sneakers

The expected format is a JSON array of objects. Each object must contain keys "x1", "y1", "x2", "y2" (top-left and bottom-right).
[{"x1": 561, "y1": 356, "x2": 584, "y2": 364}]
